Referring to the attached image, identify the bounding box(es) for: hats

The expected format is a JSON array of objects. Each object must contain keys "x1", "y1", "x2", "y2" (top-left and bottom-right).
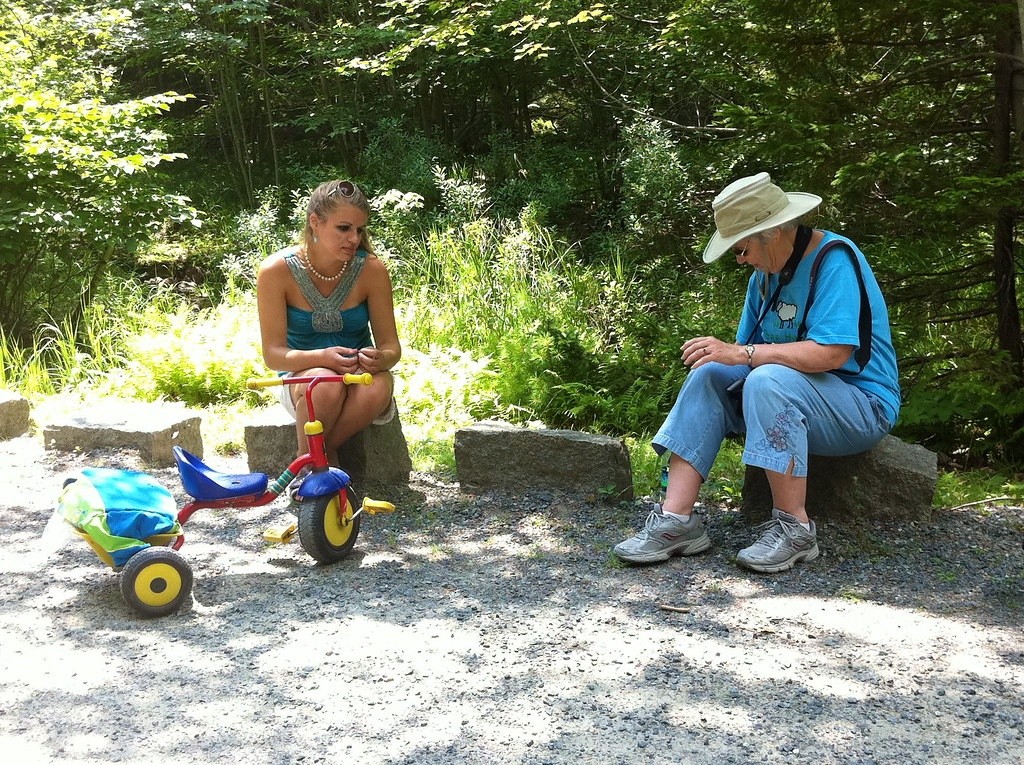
[{"x1": 703, "y1": 171, "x2": 823, "y2": 264}]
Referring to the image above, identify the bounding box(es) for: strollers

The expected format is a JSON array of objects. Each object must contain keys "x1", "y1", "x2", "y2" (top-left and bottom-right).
[{"x1": 58, "y1": 372, "x2": 399, "y2": 621}]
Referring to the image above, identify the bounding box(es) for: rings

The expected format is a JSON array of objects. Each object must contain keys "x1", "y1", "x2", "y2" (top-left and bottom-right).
[{"x1": 703, "y1": 347, "x2": 708, "y2": 355}]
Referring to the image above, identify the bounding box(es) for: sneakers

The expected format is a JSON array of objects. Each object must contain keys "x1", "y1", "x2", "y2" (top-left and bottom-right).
[
  {"x1": 613, "y1": 504, "x2": 712, "y2": 565},
  {"x1": 735, "y1": 508, "x2": 819, "y2": 573}
]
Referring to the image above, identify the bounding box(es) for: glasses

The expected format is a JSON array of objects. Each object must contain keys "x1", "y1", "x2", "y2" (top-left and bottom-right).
[
  {"x1": 731, "y1": 235, "x2": 756, "y2": 257},
  {"x1": 312, "y1": 180, "x2": 367, "y2": 210}
]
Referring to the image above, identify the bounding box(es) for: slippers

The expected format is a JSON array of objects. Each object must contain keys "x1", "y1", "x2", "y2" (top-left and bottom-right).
[{"x1": 291, "y1": 473, "x2": 307, "y2": 503}]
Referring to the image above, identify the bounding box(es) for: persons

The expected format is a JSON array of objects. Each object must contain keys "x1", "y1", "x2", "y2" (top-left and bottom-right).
[
  {"x1": 257, "y1": 179, "x2": 401, "y2": 505},
  {"x1": 613, "y1": 168, "x2": 902, "y2": 575}
]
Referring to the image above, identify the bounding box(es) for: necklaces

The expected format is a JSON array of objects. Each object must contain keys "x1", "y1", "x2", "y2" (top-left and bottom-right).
[{"x1": 304, "y1": 242, "x2": 347, "y2": 281}]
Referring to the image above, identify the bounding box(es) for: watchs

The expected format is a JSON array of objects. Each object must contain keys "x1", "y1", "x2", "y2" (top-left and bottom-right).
[{"x1": 745, "y1": 342, "x2": 755, "y2": 367}]
